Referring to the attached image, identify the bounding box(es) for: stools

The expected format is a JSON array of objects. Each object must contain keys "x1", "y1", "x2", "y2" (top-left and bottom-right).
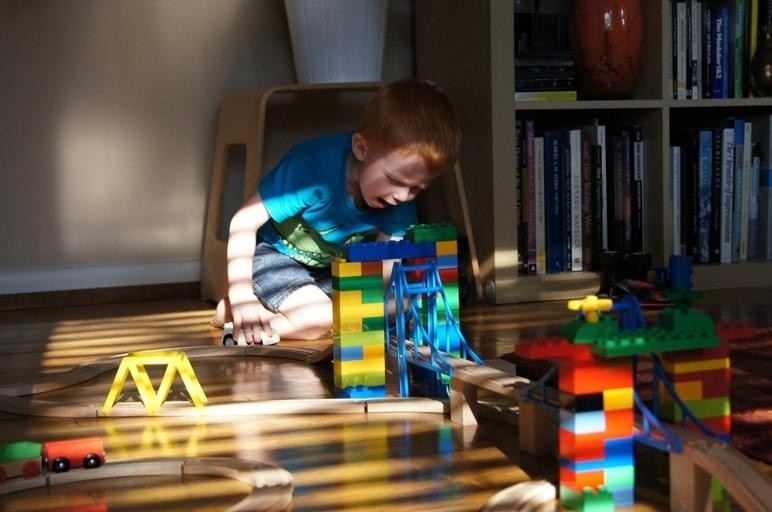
[{"x1": 200, "y1": 82, "x2": 484, "y2": 304}]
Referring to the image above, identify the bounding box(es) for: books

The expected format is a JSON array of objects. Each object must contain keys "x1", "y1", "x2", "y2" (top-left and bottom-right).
[
  {"x1": 669, "y1": 118, "x2": 766, "y2": 264},
  {"x1": 515, "y1": 61, "x2": 578, "y2": 102},
  {"x1": 515, "y1": 119, "x2": 661, "y2": 275},
  {"x1": 671, "y1": 0, "x2": 758, "y2": 102}
]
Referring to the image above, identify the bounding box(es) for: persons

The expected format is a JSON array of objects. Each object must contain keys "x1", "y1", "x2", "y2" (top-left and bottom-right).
[{"x1": 208, "y1": 77, "x2": 463, "y2": 343}]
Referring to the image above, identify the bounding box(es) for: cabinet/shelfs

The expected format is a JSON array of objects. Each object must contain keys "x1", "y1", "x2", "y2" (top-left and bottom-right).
[{"x1": 411, "y1": 0, "x2": 771, "y2": 307}]
